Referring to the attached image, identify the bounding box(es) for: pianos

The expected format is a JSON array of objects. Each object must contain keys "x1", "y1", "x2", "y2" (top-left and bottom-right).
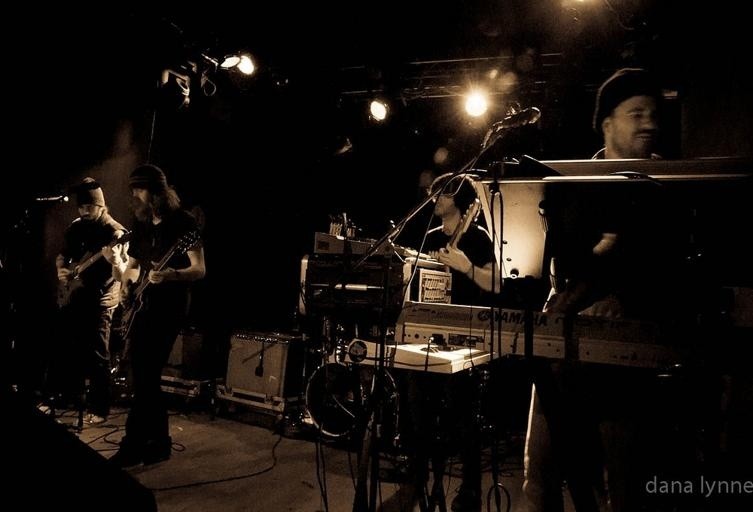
[{"x1": 394, "y1": 300, "x2": 753, "y2": 376}]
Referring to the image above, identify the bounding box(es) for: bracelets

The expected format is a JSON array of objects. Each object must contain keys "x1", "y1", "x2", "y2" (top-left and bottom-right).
[
  {"x1": 464, "y1": 264, "x2": 474, "y2": 276},
  {"x1": 174, "y1": 270, "x2": 180, "y2": 281}
]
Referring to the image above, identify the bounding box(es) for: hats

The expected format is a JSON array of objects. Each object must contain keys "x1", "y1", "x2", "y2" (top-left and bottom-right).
[
  {"x1": 128, "y1": 164, "x2": 167, "y2": 192},
  {"x1": 75, "y1": 177, "x2": 104, "y2": 206},
  {"x1": 592, "y1": 67, "x2": 658, "y2": 125}
]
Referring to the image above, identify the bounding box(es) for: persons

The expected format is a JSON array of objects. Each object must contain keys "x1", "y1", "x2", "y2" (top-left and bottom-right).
[
  {"x1": 520, "y1": 67, "x2": 690, "y2": 512},
  {"x1": 398, "y1": 172, "x2": 504, "y2": 512},
  {"x1": 108, "y1": 164, "x2": 207, "y2": 469},
  {"x1": 54, "y1": 178, "x2": 129, "y2": 430}
]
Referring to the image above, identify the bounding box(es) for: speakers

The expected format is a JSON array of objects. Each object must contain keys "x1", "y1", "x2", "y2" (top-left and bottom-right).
[
  {"x1": 299, "y1": 256, "x2": 451, "y2": 446},
  {"x1": 224, "y1": 331, "x2": 303, "y2": 397}
]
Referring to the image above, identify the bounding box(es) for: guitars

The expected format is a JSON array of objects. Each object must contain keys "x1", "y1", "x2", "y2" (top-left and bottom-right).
[
  {"x1": 57, "y1": 230, "x2": 133, "y2": 308},
  {"x1": 121, "y1": 230, "x2": 200, "y2": 339}
]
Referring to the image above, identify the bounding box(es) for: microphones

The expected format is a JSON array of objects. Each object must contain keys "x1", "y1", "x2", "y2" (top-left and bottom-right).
[
  {"x1": 36, "y1": 195, "x2": 70, "y2": 204},
  {"x1": 494, "y1": 107, "x2": 540, "y2": 130}
]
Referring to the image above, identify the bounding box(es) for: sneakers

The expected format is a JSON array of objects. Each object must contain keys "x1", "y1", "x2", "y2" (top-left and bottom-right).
[{"x1": 104, "y1": 436, "x2": 174, "y2": 475}]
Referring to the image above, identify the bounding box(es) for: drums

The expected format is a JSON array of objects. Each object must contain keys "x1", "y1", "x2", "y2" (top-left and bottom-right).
[{"x1": 306, "y1": 362, "x2": 397, "y2": 436}]
